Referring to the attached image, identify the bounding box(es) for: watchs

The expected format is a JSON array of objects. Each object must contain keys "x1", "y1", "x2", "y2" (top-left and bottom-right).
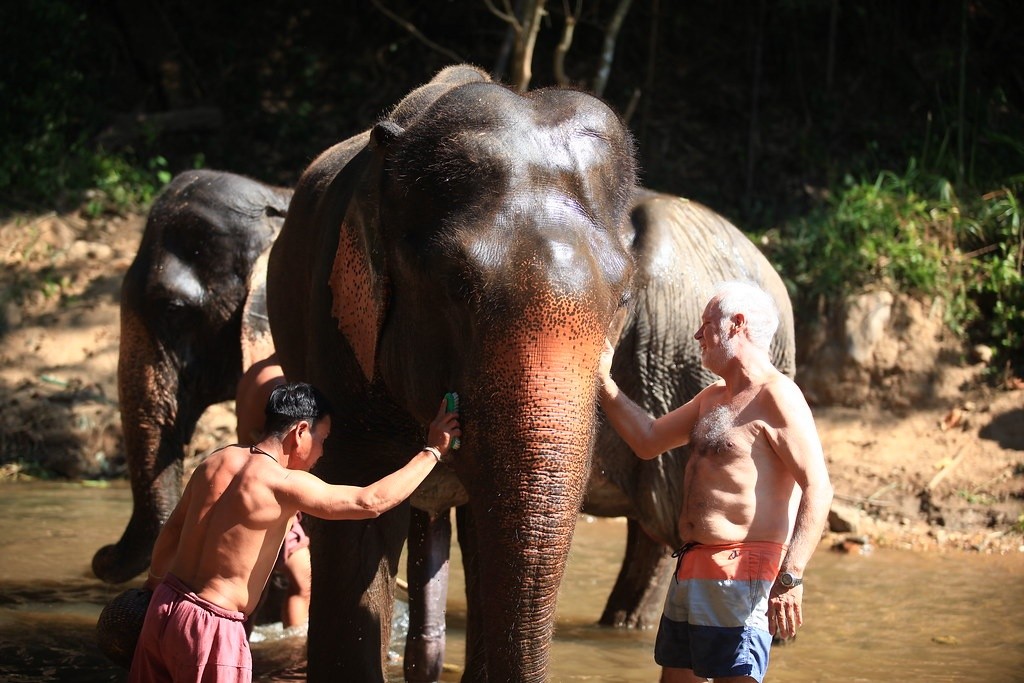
[{"x1": 777, "y1": 572, "x2": 802, "y2": 588}]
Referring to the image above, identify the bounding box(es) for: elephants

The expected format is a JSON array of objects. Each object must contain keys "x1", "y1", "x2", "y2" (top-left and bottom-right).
[{"x1": 92, "y1": 64, "x2": 796, "y2": 683}]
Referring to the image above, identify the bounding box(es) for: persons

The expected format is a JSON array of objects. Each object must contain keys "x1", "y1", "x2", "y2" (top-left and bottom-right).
[
  {"x1": 235, "y1": 354, "x2": 312, "y2": 628},
  {"x1": 597, "y1": 280, "x2": 832, "y2": 683},
  {"x1": 129, "y1": 382, "x2": 462, "y2": 683}
]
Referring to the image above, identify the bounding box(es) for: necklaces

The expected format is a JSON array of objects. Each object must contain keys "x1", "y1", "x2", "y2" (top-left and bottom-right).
[{"x1": 245, "y1": 443, "x2": 280, "y2": 467}]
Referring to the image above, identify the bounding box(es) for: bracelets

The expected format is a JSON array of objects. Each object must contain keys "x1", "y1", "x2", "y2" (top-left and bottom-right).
[
  {"x1": 148, "y1": 569, "x2": 162, "y2": 581},
  {"x1": 424, "y1": 446, "x2": 441, "y2": 461}
]
